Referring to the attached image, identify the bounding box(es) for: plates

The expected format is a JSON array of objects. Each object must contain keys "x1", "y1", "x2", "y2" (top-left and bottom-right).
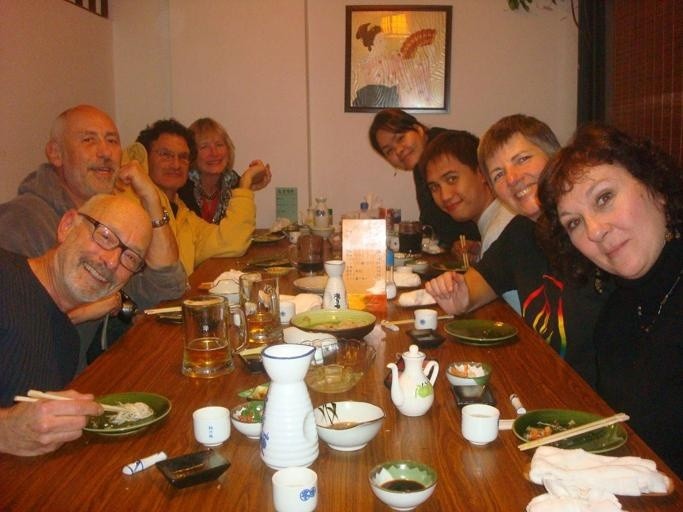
[
  {"x1": 450, "y1": 384, "x2": 497, "y2": 408},
  {"x1": 234, "y1": 343, "x2": 269, "y2": 403},
  {"x1": 431, "y1": 261, "x2": 473, "y2": 272},
  {"x1": 406, "y1": 329, "x2": 446, "y2": 348},
  {"x1": 155, "y1": 449, "x2": 229, "y2": 487},
  {"x1": 251, "y1": 230, "x2": 286, "y2": 243},
  {"x1": 442, "y1": 318, "x2": 519, "y2": 345},
  {"x1": 384, "y1": 354, "x2": 437, "y2": 391},
  {"x1": 291, "y1": 275, "x2": 327, "y2": 292},
  {"x1": 511, "y1": 409, "x2": 628, "y2": 459},
  {"x1": 155, "y1": 305, "x2": 182, "y2": 325},
  {"x1": 248, "y1": 255, "x2": 290, "y2": 271},
  {"x1": 82, "y1": 391, "x2": 171, "y2": 436}
]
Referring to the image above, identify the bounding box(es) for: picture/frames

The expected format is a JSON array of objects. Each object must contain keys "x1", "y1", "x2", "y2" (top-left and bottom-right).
[{"x1": 341, "y1": 3, "x2": 454, "y2": 116}]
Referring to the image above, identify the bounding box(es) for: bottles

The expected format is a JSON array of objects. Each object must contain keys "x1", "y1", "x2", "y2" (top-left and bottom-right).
[
  {"x1": 257, "y1": 341, "x2": 321, "y2": 469},
  {"x1": 385, "y1": 247, "x2": 396, "y2": 300},
  {"x1": 307, "y1": 196, "x2": 330, "y2": 228},
  {"x1": 321, "y1": 260, "x2": 346, "y2": 311},
  {"x1": 358, "y1": 202, "x2": 371, "y2": 219}
]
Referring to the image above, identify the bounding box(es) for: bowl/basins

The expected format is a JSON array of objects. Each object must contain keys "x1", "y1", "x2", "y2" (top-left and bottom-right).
[
  {"x1": 289, "y1": 307, "x2": 377, "y2": 340},
  {"x1": 444, "y1": 360, "x2": 493, "y2": 388},
  {"x1": 311, "y1": 399, "x2": 385, "y2": 454},
  {"x1": 368, "y1": 459, "x2": 439, "y2": 511},
  {"x1": 298, "y1": 336, "x2": 376, "y2": 393},
  {"x1": 393, "y1": 253, "x2": 428, "y2": 271},
  {"x1": 311, "y1": 226, "x2": 335, "y2": 238},
  {"x1": 226, "y1": 402, "x2": 267, "y2": 444}
]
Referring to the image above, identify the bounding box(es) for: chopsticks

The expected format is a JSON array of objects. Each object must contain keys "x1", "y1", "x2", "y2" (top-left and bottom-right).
[
  {"x1": 12, "y1": 388, "x2": 127, "y2": 413},
  {"x1": 514, "y1": 412, "x2": 630, "y2": 450},
  {"x1": 387, "y1": 314, "x2": 455, "y2": 326},
  {"x1": 143, "y1": 306, "x2": 182, "y2": 315},
  {"x1": 459, "y1": 234, "x2": 469, "y2": 269}
]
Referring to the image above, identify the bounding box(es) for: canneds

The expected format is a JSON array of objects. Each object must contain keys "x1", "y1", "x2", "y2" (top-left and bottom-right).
[{"x1": 378, "y1": 207, "x2": 394, "y2": 230}]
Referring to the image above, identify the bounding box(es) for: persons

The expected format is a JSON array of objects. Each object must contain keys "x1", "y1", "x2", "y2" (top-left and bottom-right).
[
  {"x1": 1, "y1": 104, "x2": 188, "y2": 373},
  {"x1": 369, "y1": 109, "x2": 481, "y2": 250},
  {"x1": 0, "y1": 192, "x2": 152, "y2": 457},
  {"x1": 535, "y1": 121, "x2": 683, "y2": 477},
  {"x1": 420, "y1": 128, "x2": 522, "y2": 317},
  {"x1": 425, "y1": 113, "x2": 592, "y2": 370},
  {"x1": 352, "y1": 21, "x2": 429, "y2": 107},
  {"x1": 134, "y1": 114, "x2": 272, "y2": 281}
]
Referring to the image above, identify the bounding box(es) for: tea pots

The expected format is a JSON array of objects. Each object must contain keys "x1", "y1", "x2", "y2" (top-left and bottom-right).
[
  {"x1": 398, "y1": 221, "x2": 434, "y2": 255},
  {"x1": 383, "y1": 344, "x2": 440, "y2": 420},
  {"x1": 181, "y1": 294, "x2": 248, "y2": 379},
  {"x1": 237, "y1": 271, "x2": 282, "y2": 344},
  {"x1": 287, "y1": 234, "x2": 325, "y2": 277}
]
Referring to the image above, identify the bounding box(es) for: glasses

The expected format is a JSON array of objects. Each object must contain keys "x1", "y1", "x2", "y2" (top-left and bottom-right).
[{"x1": 76, "y1": 212, "x2": 147, "y2": 273}]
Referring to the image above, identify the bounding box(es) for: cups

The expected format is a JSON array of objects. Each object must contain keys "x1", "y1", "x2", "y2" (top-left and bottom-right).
[
  {"x1": 191, "y1": 405, "x2": 230, "y2": 448},
  {"x1": 277, "y1": 300, "x2": 293, "y2": 324},
  {"x1": 412, "y1": 309, "x2": 438, "y2": 331},
  {"x1": 289, "y1": 231, "x2": 301, "y2": 244},
  {"x1": 271, "y1": 467, "x2": 317, "y2": 511},
  {"x1": 460, "y1": 403, "x2": 501, "y2": 447}
]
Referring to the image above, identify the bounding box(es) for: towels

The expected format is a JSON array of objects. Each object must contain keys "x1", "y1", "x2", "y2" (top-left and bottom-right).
[
  {"x1": 202, "y1": 269, "x2": 249, "y2": 285},
  {"x1": 523, "y1": 441, "x2": 675, "y2": 498},
  {"x1": 390, "y1": 268, "x2": 421, "y2": 289},
  {"x1": 279, "y1": 324, "x2": 340, "y2": 360},
  {"x1": 258, "y1": 287, "x2": 325, "y2": 317},
  {"x1": 525, "y1": 490, "x2": 624, "y2": 512},
  {"x1": 397, "y1": 287, "x2": 438, "y2": 307}
]
